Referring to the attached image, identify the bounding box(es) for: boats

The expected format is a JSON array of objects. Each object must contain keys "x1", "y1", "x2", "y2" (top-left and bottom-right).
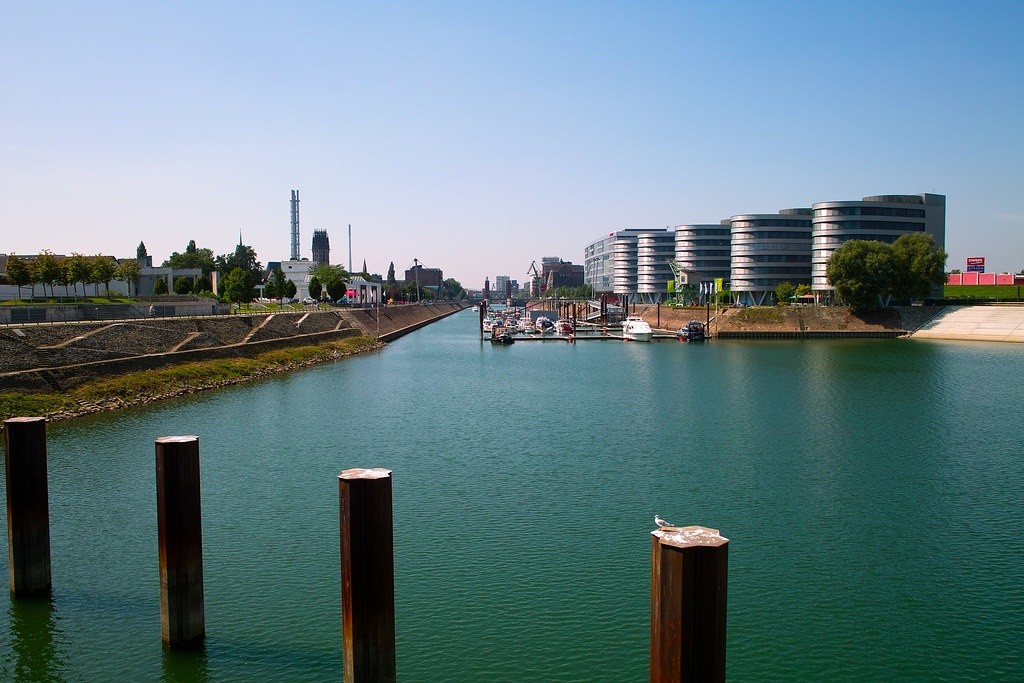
[
  {"x1": 677, "y1": 321, "x2": 705, "y2": 343},
  {"x1": 472, "y1": 306, "x2": 478, "y2": 312},
  {"x1": 482, "y1": 305, "x2": 574, "y2": 345},
  {"x1": 621, "y1": 313, "x2": 654, "y2": 341}
]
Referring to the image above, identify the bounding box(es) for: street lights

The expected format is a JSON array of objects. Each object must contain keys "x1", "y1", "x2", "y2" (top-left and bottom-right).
[{"x1": 260, "y1": 279, "x2": 264, "y2": 303}]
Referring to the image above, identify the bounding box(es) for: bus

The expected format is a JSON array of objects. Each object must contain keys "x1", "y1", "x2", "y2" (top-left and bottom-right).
[{"x1": 789, "y1": 295, "x2": 816, "y2": 307}]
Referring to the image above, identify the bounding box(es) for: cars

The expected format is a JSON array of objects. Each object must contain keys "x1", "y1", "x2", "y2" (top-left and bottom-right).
[{"x1": 303, "y1": 297, "x2": 313, "y2": 304}]
